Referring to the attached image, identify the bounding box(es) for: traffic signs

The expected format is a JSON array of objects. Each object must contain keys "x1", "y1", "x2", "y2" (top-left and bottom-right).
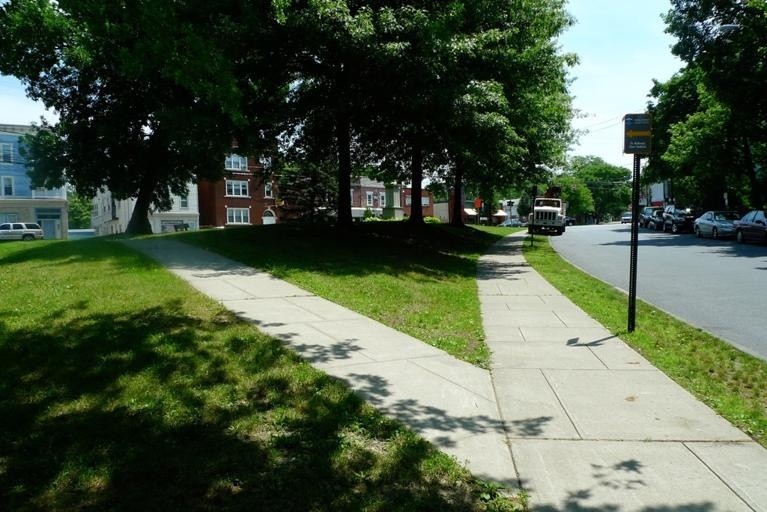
[{"x1": 623, "y1": 114, "x2": 652, "y2": 156}]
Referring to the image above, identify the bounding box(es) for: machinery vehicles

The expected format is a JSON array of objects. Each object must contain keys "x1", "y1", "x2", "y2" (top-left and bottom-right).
[{"x1": 527, "y1": 186, "x2": 566, "y2": 235}]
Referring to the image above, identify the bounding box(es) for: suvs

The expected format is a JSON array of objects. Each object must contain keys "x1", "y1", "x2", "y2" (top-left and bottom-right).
[
  {"x1": 661, "y1": 203, "x2": 702, "y2": 234},
  {"x1": 639, "y1": 205, "x2": 663, "y2": 228}
]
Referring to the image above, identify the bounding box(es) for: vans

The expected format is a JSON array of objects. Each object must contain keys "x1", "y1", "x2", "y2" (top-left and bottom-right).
[{"x1": 0, "y1": 222, "x2": 44, "y2": 241}]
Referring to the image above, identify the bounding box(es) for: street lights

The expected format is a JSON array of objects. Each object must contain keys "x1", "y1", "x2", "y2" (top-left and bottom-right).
[{"x1": 668, "y1": 177, "x2": 675, "y2": 204}]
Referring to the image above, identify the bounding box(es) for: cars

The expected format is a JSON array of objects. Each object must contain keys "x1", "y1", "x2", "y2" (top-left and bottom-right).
[
  {"x1": 731, "y1": 209, "x2": 767, "y2": 244},
  {"x1": 648, "y1": 208, "x2": 664, "y2": 231},
  {"x1": 479, "y1": 216, "x2": 576, "y2": 226},
  {"x1": 620, "y1": 212, "x2": 633, "y2": 224},
  {"x1": 693, "y1": 211, "x2": 741, "y2": 240}
]
